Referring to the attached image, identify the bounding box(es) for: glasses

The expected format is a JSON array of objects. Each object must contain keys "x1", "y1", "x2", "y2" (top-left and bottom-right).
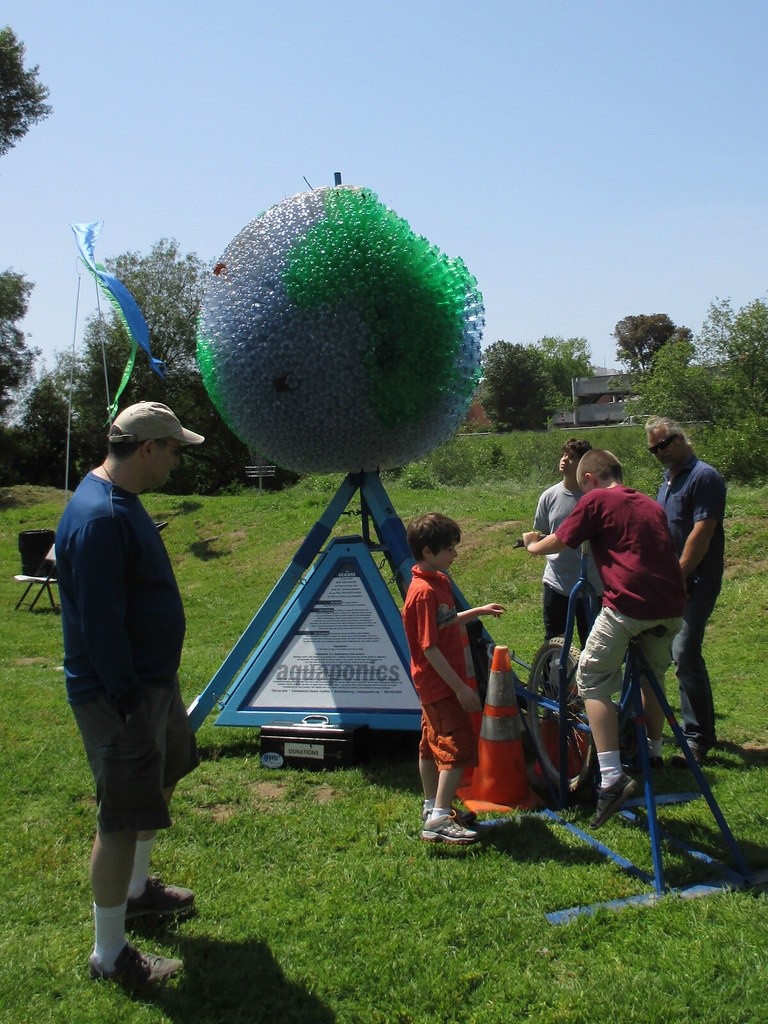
[
  {"x1": 648, "y1": 435, "x2": 675, "y2": 454},
  {"x1": 155, "y1": 439, "x2": 186, "y2": 458}
]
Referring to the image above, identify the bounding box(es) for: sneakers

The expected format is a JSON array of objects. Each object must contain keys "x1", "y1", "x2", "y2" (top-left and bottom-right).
[
  {"x1": 89, "y1": 942, "x2": 185, "y2": 982},
  {"x1": 421, "y1": 806, "x2": 476, "y2": 825},
  {"x1": 421, "y1": 809, "x2": 478, "y2": 844},
  {"x1": 650, "y1": 756, "x2": 665, "y2": 772},
  {"x1": 590, "y1": 774, "x2": 637, "y2": 830},
  {"x1": 125, "y1": 871, "x2": 197, "y2": 919},
  {"x1": 672, "y1": 746, "x2": 708, "y2": 767}
]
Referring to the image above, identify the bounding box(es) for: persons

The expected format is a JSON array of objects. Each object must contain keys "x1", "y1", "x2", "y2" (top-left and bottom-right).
[
  {"x1": 402, "y1": 512, "x2": 507, "y2": 844},
  {"x1": 533, "y1": 439, "x2": 603, "y2": 697},
  {"x1": 522, "y1": 449, "x2": 688, "y2": 831},
  {"x1": 55, "y1": 401, "x2": 205, "y2": 986},
  {"x1": 646, "y1": 416, "x2": 727, "y2": 763}
]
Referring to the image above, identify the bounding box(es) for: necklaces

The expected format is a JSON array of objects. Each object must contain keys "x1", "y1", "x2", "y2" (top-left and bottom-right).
[{"x1": 102, "y1": 463, "x2": 118, "y2": 484}]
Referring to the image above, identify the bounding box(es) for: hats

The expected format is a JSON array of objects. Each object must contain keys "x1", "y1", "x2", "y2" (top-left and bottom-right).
[{"x1": 107, "y1": 400, "x2": 206, "y2": 444}]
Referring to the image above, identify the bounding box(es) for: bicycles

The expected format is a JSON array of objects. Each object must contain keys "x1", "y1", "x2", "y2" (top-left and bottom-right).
[{"x1": 512, "y1": 533, "x2": 768, "y2": 927}]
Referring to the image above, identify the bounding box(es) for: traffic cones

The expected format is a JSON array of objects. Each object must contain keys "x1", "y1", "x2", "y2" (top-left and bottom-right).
[
  {"x1": 533, "y1": 686, "x2": 589, "y2": 781},
  {"x1": 455, "y1": 646, "x2": 545, "y2": 814}
]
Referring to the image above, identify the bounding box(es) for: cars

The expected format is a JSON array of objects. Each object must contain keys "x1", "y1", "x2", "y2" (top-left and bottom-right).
[{"x1": 617, "y1": 414, "x2": 652, "y2": 425}]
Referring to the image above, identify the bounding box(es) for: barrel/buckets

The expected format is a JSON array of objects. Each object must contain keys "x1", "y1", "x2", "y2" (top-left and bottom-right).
[{"x1": 18, "y1": 529, "x2": 56, "y2": 577}]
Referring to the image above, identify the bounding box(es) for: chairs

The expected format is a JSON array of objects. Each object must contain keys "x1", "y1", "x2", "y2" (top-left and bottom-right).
[{"x1": 13, "y1": 544, "x2": 57, "y2": 611}]
